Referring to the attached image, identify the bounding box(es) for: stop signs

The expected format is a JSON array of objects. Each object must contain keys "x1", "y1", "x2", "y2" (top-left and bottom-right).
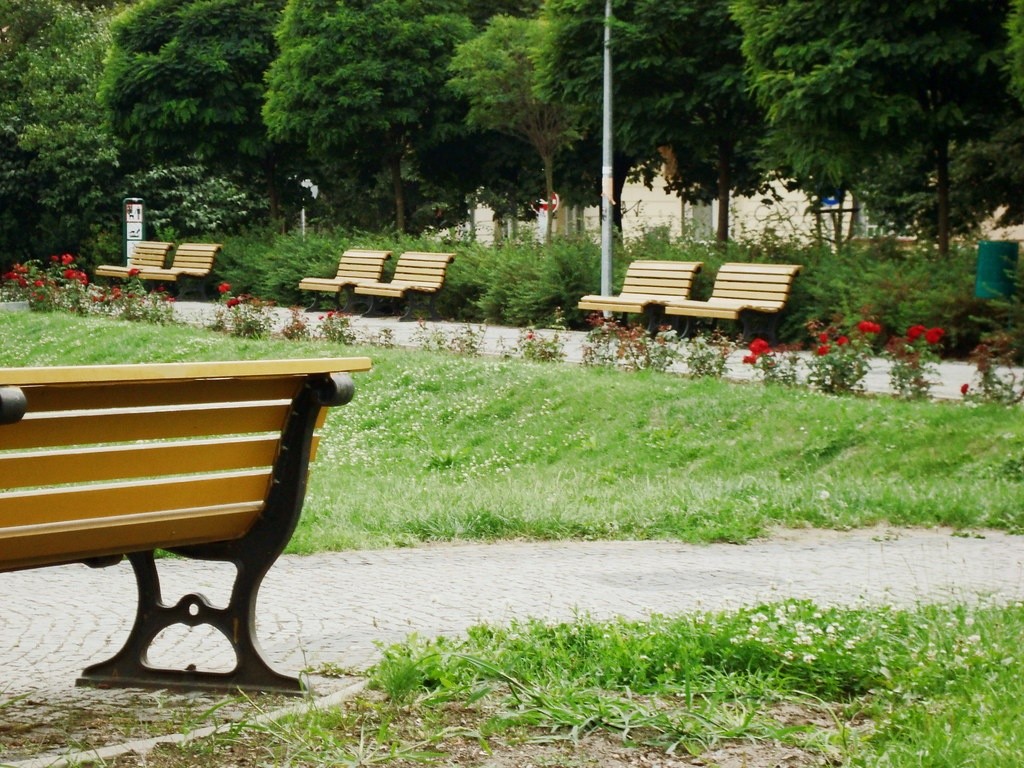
[{"x1": 531, "y1": 190, "x2": 559, "y2": 216}]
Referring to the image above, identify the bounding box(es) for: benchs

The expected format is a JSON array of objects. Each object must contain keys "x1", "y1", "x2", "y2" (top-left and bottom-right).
[
  {"x1": 577, "y1": 259, "x2": 703, "y2": 338},
  {"x1": 0, "y1": 356, "x2": 374, "y2": 700},
  {"x1": 138, "y1": 243, "x2": 223, "y2": 301},
  {"x1": 94, "y1": 241, "x2": 175, "y2": 290},
  {"x1": 665, "y1": 262, "x2": 804, "y2": 347},
  {"x1": 354, "y1": 251, "x2": 458, "y2": 322},
  {"x1": 299, "y1": 248, "x2": 393, "y2": 317}
]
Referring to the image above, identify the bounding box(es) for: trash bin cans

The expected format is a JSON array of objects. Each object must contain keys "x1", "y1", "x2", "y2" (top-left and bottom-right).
[{"x1": 975, "y1": 240, "x2": 1019, "y2": 297}]
